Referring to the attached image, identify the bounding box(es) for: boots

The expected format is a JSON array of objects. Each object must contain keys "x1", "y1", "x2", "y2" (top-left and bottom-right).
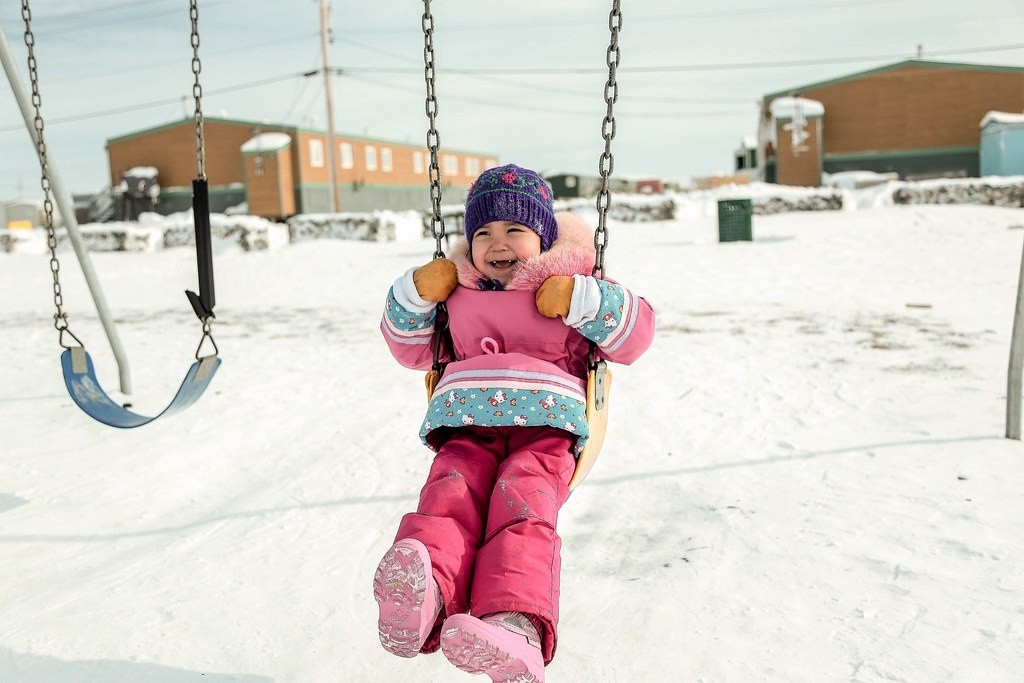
[
  {"x1": 372, "y1": 538, "x2": 445, "y2": 658},
  {"x1": 440, "y1": 611, "x2": 546, "y2": 683}
]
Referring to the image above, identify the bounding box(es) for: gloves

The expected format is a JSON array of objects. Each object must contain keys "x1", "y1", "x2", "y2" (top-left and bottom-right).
[
  {"x1": 535, "y1": 275, "x2": 575, "y2": 319},
  {"x1": 413, "y1": 258, "x2": 458, "y2": 303}
]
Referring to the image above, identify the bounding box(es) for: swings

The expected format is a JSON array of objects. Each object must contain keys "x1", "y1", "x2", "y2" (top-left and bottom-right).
[
  {"x1": 420, "y1": 0, "x2": 626, "y2": 502},
  {"x1": 19, "y1": 0, "x2": 224, "y2": 432}
]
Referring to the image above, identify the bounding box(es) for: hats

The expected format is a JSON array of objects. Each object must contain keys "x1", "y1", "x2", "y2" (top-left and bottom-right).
[{"x1": 463, "y1": 163, "x2": 558, "y2": 252}]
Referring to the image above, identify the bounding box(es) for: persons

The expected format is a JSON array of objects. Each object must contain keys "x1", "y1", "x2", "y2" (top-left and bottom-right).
[{"x1": 371, "y1": 164, "x2": 655, "y2": 683}]
